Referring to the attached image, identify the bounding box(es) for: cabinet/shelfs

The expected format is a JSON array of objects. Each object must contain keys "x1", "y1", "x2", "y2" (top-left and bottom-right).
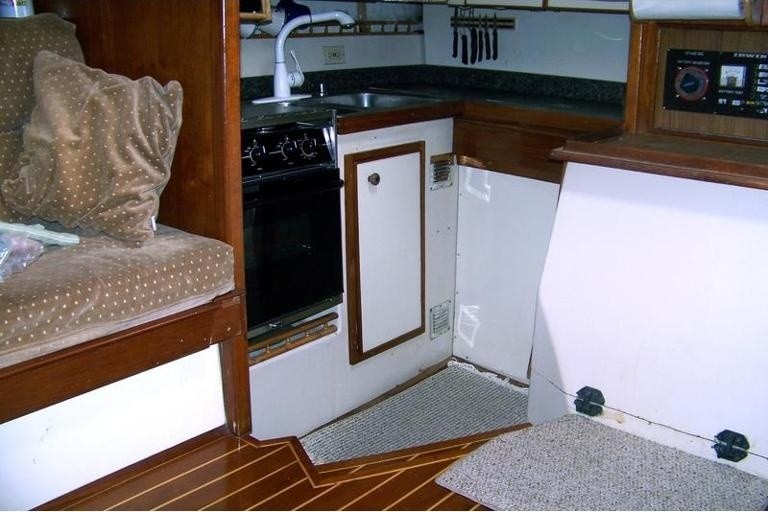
[{"x1": 342, "y1": 138, "x2": 428, "y2": 369}]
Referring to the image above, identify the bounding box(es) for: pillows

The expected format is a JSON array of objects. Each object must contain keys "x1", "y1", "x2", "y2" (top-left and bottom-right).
[
  {"x1": 1, "y1": 46, "x2": 190, "y2": 250},
  {"x1": 1, "y1": 10, "x2": 84, "y2": 224}
]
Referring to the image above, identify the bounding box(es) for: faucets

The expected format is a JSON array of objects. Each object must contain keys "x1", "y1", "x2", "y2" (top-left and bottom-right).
[{"x1": 273, "y1": 10, "x2": 356, "y2": 97}]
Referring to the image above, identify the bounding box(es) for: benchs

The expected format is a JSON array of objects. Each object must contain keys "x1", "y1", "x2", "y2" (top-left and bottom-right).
[{"x1": 0, "y1": 217, "x2": 238, "y2": 510}]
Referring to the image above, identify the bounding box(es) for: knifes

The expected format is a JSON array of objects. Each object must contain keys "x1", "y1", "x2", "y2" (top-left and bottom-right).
[{"x1": 450, "y1": 5, "x2": 499, "y2": 65}]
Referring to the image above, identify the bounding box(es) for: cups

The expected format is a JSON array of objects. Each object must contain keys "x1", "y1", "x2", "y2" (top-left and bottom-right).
[{"x1": 239, "y1": 6, "x2": 286, "y2": 39}]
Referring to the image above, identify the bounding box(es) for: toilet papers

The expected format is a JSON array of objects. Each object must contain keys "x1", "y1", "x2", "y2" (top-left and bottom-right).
[{"x1": 632, "y1": 0, "x2": 750, "y2": 22}]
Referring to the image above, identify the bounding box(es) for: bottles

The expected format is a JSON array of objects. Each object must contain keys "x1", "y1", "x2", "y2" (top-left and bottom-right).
[{"x1": 0, "y1": 0, "x2": 35, "y2": 19}]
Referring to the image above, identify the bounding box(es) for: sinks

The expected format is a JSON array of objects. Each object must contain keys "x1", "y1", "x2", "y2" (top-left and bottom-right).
[{"x1": 290, "y1": 87, "x2": 460, "y2": 111}]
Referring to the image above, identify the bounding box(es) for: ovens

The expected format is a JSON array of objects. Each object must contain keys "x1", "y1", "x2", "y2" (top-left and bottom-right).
[{"x1": 224, "y1": 102, "x2": 344, "y2": 340}]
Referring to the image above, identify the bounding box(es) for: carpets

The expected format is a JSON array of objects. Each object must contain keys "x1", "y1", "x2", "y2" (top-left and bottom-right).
[
  {"x1": 297, "y1": 360, "x2": 529, "y2": 469},
  {"x1": 432, "y1": 412, "x2": 767, "y2": 511}
]
